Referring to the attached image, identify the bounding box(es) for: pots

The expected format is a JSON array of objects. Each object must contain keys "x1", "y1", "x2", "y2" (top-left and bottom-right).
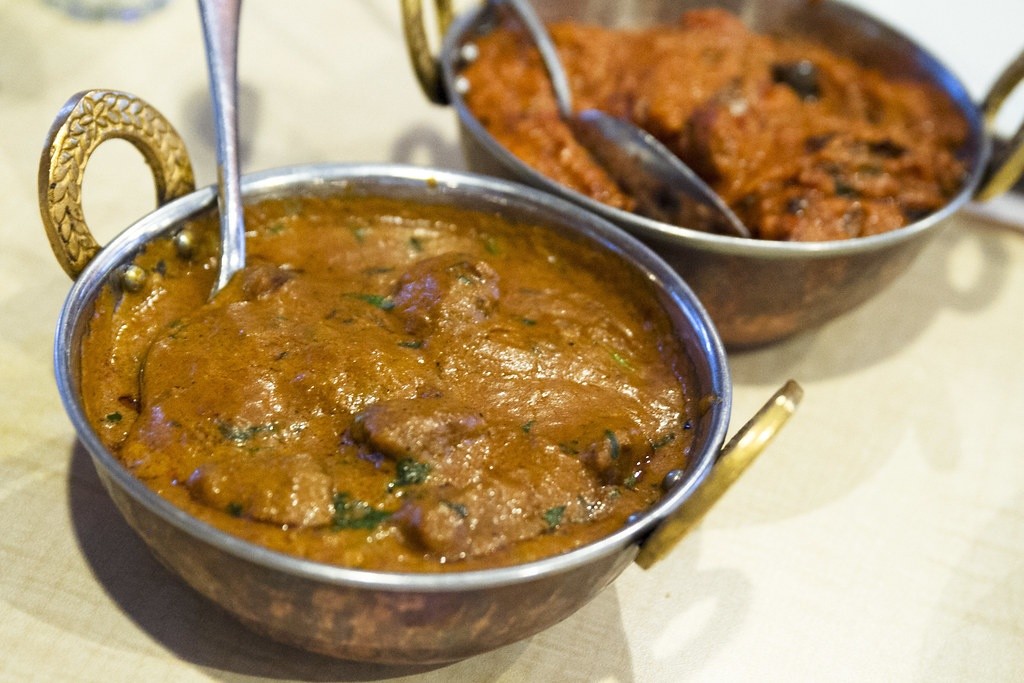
[
  {"x1": 399, "y1": 0, "x2": 1024, "y2": 357},
  {"x1": 37, "y1": 86, "x2": 804, "y2": 666}
]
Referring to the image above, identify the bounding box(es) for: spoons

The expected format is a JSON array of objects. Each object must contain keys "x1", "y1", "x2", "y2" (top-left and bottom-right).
[{"x1": 510, "y1": 1, "x2": 751, "y2": 242}]
